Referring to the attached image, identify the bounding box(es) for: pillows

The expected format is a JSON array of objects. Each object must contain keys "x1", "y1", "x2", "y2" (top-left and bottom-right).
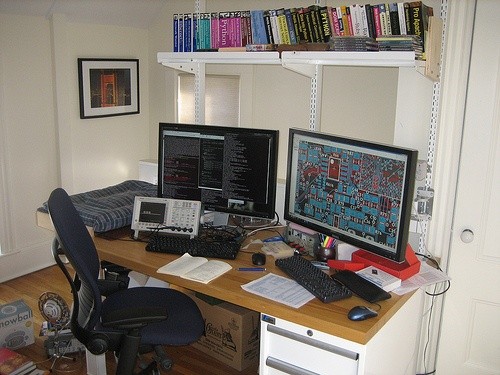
[{"x1": 38, "y1": 179, "x2": 158, "y2": 232}]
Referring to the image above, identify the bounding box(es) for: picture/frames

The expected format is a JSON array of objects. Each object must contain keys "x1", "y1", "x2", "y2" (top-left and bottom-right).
[{"x1": 77, "y1": 58, "x2": 140, "y2": 119}]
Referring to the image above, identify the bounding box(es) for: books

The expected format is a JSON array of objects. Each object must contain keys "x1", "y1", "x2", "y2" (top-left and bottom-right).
[
  {"x1": 0, "y1": 347, "x2": 37, "y2": 375},
  {"x1": 156, "y1": 253, "x2": 232, "y2": 284},
  {"x1": 173, "y1": 1, "x2": 434, "y2": 61}
]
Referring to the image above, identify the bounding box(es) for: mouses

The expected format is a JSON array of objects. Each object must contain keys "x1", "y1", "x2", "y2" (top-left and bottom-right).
[
  {"x1": 252, "y1": 253, "x2": 266, "y2": 265},
  {"x1": 348, "y1": 306, "x2": 377, "y2": 320}
]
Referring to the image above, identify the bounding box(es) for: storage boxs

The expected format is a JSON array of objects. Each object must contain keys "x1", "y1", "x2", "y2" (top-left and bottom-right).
[
  {"x1": 170, "y1": 284, "x2": 260, "y2": 372},
  {"x1": 0, "y1": 300, "x2": 35, "y2": 349}
]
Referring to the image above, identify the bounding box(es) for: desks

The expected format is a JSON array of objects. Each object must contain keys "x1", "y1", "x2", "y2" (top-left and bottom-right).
[{"x1": 36, "y1": 180, "x2": 440, "y2": 375}]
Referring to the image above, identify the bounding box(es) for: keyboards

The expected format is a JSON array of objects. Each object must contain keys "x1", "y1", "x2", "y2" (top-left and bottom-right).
[
  {"x1": 276, "y1": 255, "x2": 353, "y2": 301},
  {"x1": 146, "y1": 234, "x2": 241, "y2": 260}
]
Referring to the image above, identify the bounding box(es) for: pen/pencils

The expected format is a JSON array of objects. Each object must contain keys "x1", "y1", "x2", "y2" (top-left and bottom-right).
[
  {"x1": 318, "y1": 232, "x2": 338, "y2": 248},
  {"x1": 236, "y1": 268, "x2": 266, "y2": 271}
]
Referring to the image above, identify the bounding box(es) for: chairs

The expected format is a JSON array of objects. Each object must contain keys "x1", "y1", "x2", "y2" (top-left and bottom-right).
[{"x1": 47, "y1": 188, "x2": 204, "y2": 375}]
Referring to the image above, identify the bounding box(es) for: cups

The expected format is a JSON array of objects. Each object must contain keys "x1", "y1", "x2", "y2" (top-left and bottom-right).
[{"x1": 316, "y1": 245, "x2": 334, "y2": 262}]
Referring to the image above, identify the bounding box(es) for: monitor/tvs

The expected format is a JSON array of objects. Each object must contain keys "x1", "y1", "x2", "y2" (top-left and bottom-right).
[
  {"x1": 158, "y1": 122, "x2": 278, "y2": 219},
  {"x1": 283, "y1": 126, "x2": 418, "y2": 263}
]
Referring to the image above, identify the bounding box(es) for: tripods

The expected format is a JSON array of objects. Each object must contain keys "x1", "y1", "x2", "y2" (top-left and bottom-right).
[{"x1": 34, "y1": 323, "x2": 76, "y2": 373}]
